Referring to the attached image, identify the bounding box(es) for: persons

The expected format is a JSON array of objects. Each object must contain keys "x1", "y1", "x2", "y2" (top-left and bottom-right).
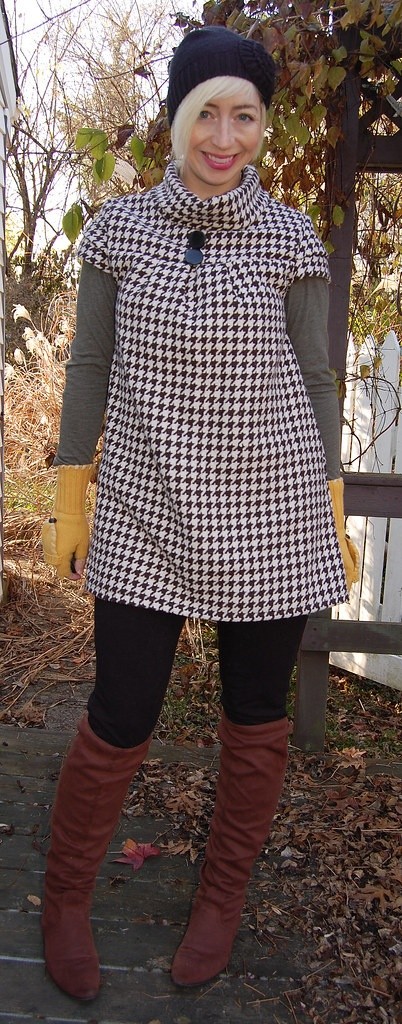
[{"x1": 41, "y1": 25, "x2": 359, "y2": 1001}]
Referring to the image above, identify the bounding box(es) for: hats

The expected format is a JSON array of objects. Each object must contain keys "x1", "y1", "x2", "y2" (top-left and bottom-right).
[{"x1": 167, "y1": 26, "x2": 276, "y2": 127}]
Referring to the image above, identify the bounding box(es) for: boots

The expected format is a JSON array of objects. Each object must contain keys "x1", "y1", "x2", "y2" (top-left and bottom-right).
[
  {"x1": 40, "y1": 712, "x2": 152, "y2": 1002},
  {"x1": 170, "y1": 709, "x2": 291, "y2": 987}
]
